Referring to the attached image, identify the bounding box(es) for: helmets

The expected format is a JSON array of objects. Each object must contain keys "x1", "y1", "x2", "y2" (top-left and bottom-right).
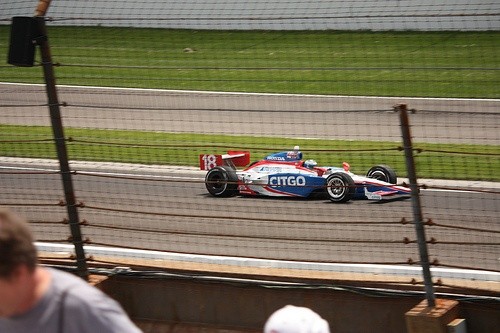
[{"x1": 302, "y1": 159, "x2": 318, "y2": 172}]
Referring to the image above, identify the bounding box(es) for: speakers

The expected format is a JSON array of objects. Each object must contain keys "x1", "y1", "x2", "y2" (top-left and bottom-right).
[{"x1": 8, "y1": 17, "x2": 39, "y2": 68}]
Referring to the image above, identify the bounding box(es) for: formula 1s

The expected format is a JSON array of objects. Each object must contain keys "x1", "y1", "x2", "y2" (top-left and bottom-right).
[{"x1": 199, "y1": 145, "x2": 430, "y2": 204}]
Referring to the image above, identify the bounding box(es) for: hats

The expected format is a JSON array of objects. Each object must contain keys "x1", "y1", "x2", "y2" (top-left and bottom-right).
[{"x1": 263, "y1": 304, "x2": 330, "y2": 333}]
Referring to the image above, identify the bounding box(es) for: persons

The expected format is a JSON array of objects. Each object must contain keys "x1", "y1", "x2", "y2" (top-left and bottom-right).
[
  {"x1": 0, "y1": 209, "x2": 144, "y2": 333},
  {"x1": 303, "y1": 159, "x2": 317, "y2": 169},
  {"x1": 265, "y1": 304, "x2": 330, "y2": 333}
]
